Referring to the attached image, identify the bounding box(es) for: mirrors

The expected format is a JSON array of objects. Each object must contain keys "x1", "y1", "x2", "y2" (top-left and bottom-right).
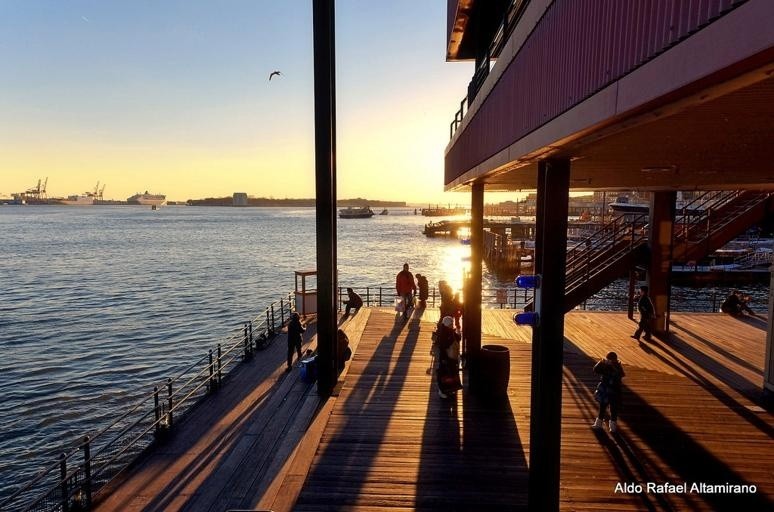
[{"x1": 268, "y1": 71, "x2": 285, "y2": 82}]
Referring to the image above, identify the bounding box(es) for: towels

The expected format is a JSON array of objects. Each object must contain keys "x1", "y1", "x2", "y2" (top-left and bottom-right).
[{"x1": 479, "y1": 345, "x2": 510, "y2": 396}]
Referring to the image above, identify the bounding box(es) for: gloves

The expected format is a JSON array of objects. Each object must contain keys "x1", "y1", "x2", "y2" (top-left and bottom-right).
[
  {"x1": 669, "y1": 235, "x2": 774, "y2": 287},
  {"x1": 337, "y1": 203, "x2": 374, "y2": 219},
  {"x1": 47, "y1": 192, "x2": 94, "y2": 205},
  {"x1": 0, "y1": 177, "x2": 54, "y2": 205},
  {"x1": 608, "y1": 199, "x2": 704, "y2": 223}
]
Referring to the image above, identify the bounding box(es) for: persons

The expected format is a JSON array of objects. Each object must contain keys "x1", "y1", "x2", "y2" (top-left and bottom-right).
[
  {"x1": 343, "y1": 288, "x2": 363, "y2": 316},
  {"x1": 288, "y1": 313, "x2": 306, "y2": 369},
  {"x1": 630, "y1": 286, "x2": 655, "y2": 340},
  {"x1": 396, "y1": 263, "x2": 464, "y2": 399},
  {"x1": 725, "y1": 290, "x2": 756, "y2": 315},
  {"x1": 592, "y1": 352, "x2": 625, "y2": 432}
]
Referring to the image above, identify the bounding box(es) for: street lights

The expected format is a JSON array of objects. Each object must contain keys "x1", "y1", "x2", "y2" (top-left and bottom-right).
[{"x1": 436, "y1": 368, "x2": 463, "y2": 395}]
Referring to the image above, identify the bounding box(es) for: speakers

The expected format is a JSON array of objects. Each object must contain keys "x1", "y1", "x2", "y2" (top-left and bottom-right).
[{"x1": 590, "y1": 417, "x2": 619, "y2": 435}]
